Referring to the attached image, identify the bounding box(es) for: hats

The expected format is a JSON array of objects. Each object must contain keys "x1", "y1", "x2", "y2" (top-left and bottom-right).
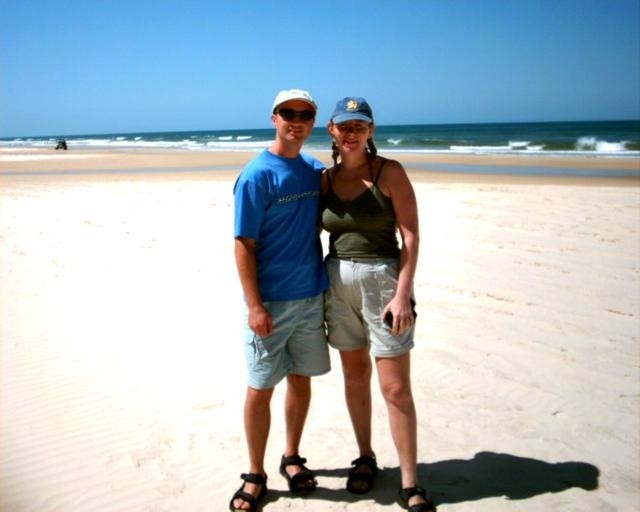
[
  {"x1": 272, "y1": 88, "x2": 319, "y2": 113},
  {"x1": 329, "y1": 96, "x2": 374, "y2": 125}
]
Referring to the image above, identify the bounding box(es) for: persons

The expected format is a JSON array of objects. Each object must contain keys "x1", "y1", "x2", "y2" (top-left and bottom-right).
[
  {"x1": 229, "y1": 87, "x2": 327, "y2": 511},
  {"x1": 318, "y1": 95, "x2": 437, "y2": 511}
]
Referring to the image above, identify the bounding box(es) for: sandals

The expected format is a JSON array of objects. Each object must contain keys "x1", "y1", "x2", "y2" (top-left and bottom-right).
[
  {"x1": 347, "y1": 456, "x2": 379, "y2": 495},
  {"x1": 278, "y1": 454, "x2": 318, "y2": 495},
  {"x1": 229, "y1": 471, "x2": 270, "y2": 510},
  {"x1": 396, "y1": 484, "x2": 437, "y2": 512}
]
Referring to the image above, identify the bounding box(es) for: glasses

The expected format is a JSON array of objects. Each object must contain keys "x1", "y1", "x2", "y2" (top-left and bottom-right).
[{"x1": 277, "y1": 108, "x2": 314, "y2": 122}]
